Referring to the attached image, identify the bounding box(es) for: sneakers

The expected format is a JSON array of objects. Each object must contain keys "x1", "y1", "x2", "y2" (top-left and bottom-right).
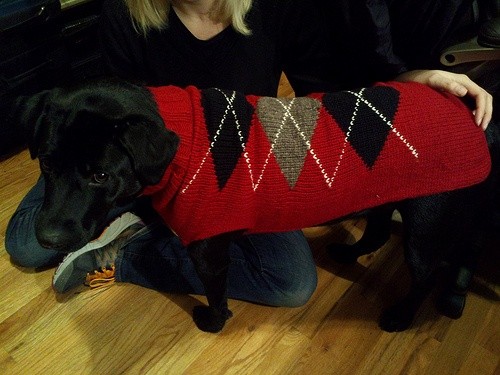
[{"x1": 52, "y1": 211, "x2": 148, "y2": 295}]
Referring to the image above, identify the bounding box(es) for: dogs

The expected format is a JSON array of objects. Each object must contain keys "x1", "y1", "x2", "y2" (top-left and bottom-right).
[{"x1": 20, "y1": 78, "x2": 500, "y2": 334}]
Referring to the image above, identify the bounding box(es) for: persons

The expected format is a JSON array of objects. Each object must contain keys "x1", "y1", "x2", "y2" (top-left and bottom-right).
[{"x1": 4, "y1": 1, "x2": 494, "y2": 307}]
